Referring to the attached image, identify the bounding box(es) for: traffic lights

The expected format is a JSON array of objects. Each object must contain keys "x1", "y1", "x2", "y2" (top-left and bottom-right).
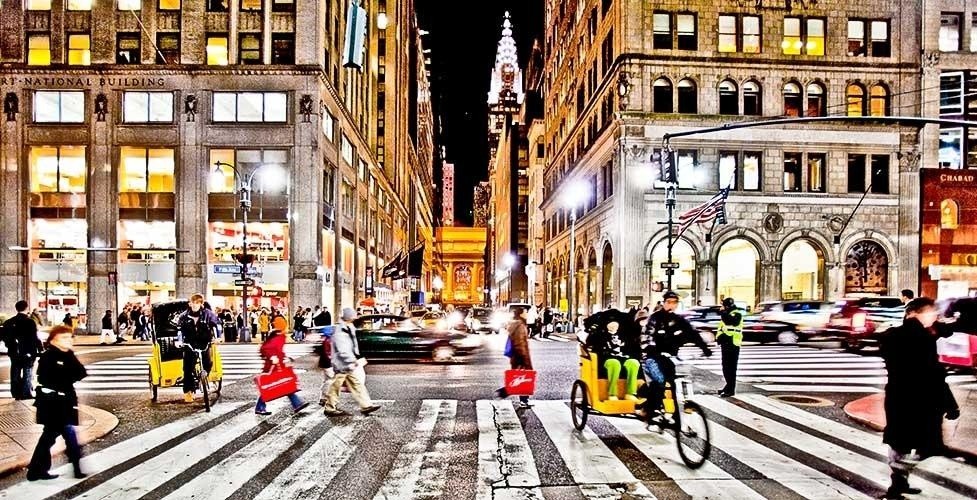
[
  {"x1": 664, "y1": 148, "x2": 678, "y2": 184},
  {"x1": 649, "y1": 147, "x2": 666, "y2": 183}
]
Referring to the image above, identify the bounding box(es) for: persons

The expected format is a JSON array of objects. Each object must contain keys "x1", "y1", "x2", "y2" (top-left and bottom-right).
[
  {"x1": 526, "y1": 298, "x2": 705, "y2": 340},
  {"x1": 645, "y1": 290, "x2": 713, "y2": 426},
  {"x1": 174, "y1": 295, "x2": 224, "y2": 405},
  {"x1": 0, "y1": 300, "x2": 43, "y2": 400},
  {"x1": 897, "y1": 286, "x2": 914, "y2": 304},
  {"x1": 100, "y1": 302, "x2": 152, "y2": 344},
  {"x1": 875, "y1": 297, "x2": 961, "y2": 500},
  {"x1": 254, "y1": 314, "x2": 309, "y2": 414},
  {"x1": 215, "y1": 303, "x2": 276, "y2": 341},
  {"x1": 25, "y1": 324, "x2": 87, "y2": 482},
  {"x1": 585, "y1": 309, "x2": 649, "y2": 405},
  {"x1": 289, "y1": 306, "x2": 331, "y2": 342},
  {"x1": 31, "y1": 308, "x2": 42, "y2": 333},
  {"x1": 319, "y1": 307, "x2": 382, "y2": 416},
  {"x1": 493, "y1": 307, "x2": 535, "y2": 410},
  {"x1": 63, "y1": 313, "x2": 73, "y2": 327},
  {"x1": 384, "y1": 304, "x2": 407, "y2": 316},
  {"x1": 714, "y1": 298, "x2": 744, "y2": 400}
]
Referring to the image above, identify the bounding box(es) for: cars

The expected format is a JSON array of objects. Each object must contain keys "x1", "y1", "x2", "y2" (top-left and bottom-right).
[
  {"x1": 313, "y1": 313, "x2": 490, "y2": 365},
  {"x1": 409, "y1": 301, "x2": 537, "y2": 335},
  {"x1": 679, "y1": 293, "x2": 976, "y2": 369},
  {"x1": 0, "y1": 311, "x2": 50, "y2": 357}
]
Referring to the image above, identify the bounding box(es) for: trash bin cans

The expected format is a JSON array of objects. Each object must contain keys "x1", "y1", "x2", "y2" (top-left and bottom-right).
[{"x1": 223, "y1": 322, "x2": 237, "y2": 342}]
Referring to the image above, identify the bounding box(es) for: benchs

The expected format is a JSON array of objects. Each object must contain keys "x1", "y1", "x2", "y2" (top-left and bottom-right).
[
  {"x1": 150, "y1": 298, "x2": 217, "y2": 362},
  {"x1": 584, "y1": 309, "x2": 650, "y2": 379}
]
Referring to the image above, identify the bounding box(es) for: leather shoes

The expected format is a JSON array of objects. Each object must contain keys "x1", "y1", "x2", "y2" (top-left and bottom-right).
[{"x1": 721, "y1": 392, "x2": 734, "y2": 397}]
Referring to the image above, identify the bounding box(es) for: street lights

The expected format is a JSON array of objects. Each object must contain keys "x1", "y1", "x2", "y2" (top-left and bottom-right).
[
  {"x1": 209, "y1": 162, "x2": 286, "y2": 342},
  {"x1": 557, "y1": 179, "x2": 592, "y2": 333},
  {"x1": 665, "y1": 186, "x2": 675, "y2": 293},
  {"x1": 502, "y1": 252, "x2": 515, "y2": 301}
]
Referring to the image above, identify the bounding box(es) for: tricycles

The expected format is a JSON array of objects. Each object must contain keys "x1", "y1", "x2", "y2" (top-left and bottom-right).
[
  {"x1": 569, "y1": 307, "x2": 710, "y2": 470},
  {"x1": 148, "y1": 298, "x2": 221, "y2": 413}
]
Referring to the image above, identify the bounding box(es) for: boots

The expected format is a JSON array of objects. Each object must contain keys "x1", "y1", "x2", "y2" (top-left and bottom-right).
[{"x1": 885, "y1": 467, "x2": 921, "y2": 500}]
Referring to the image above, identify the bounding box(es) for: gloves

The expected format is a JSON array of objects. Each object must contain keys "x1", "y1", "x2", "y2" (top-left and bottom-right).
[{"x1": 945, "y1": 407, "x2": 960, "y2": 419}]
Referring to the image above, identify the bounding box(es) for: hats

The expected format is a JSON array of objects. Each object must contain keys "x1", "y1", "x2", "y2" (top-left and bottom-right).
[
  {"x1": 273, "y1": 317, "x2": 286, "y2": 330},
  {"x1": 343, "y1": 308, "x2": 358, "y2": 321}
]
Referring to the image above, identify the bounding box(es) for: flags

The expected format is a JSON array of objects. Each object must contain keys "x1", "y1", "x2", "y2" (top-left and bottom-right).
[
  {"x1": 381, "y1": 245, "x2": 430, "y2": 281},
  {"x1": 675, "y1": 185, "x2": 728, "y2": 238}
]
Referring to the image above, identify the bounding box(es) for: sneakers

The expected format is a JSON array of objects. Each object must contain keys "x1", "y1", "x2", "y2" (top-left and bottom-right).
[
  {"x1": 184, "y1": 392, "x2": 193, "y2": 403},
  {"x1": 255, "y1": 399, "x2": 382, "y2": 415}
]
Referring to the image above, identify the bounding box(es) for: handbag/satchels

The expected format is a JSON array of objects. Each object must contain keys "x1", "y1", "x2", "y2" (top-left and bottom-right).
[
  {"x1": 253, "y1": 366, "x2": 298, "y2": 403},
  {"x1": 504, "y1": 335, "x2": 513, "y2": 358},
  {"x1": 505, "y1": 369, "x2": 537, "y2": 395}
]
